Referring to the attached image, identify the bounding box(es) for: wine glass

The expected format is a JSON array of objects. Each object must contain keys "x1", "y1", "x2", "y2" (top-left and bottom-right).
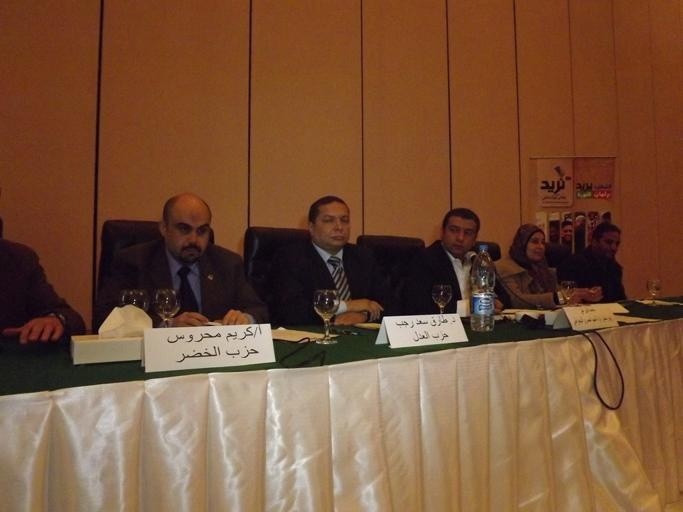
[
  {"x1": 431, "y1": 284, "x2": 452, "y2": 313},
  {"x1": 645, "y1": 280, "x2": 664, "y2": 307},
  {"x1": 312, "y1": 289, "x2": 339, "y2": 347},
  {"x1": 560, "y1": 281, "x2": 576, "y2": 304},
  {"x1": 120, "y1": 289, "x2": 178, "y2": 326}
]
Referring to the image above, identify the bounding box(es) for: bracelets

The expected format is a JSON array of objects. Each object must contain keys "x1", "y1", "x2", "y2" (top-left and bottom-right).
[{"x1": 47, "y1": 312, "x2": 67, "y2": 322}]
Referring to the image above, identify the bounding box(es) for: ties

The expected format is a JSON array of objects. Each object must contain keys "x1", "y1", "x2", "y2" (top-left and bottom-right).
[
  {"x1": 327, "y1": 255, "x2": 351, "y2": 300},
  {"x1": 177, "y1": 267, "x2": 198, "y2": 314}
]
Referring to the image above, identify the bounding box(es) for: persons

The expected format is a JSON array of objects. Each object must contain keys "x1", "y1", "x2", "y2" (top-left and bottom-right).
[
  {"x1": 261, "y1": 194, "x2": 400, "y2": 325},
  {"x1": 394, "y1": 206, "x2": 511, "y2": 313},
  {"x1": 491, "y1": 214, "x2": 628, "y2": 309},
  {"x1": 92, "y1": 190, "x2": 275, "y2": 328},
  {"x1": 0, "y1": 219, "x2": 93, "y2": 355}
]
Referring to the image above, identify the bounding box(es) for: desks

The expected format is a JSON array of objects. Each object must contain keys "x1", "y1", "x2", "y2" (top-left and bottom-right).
[{"x1": 0, "y1": 297, "x2": 681, "y2": 512}]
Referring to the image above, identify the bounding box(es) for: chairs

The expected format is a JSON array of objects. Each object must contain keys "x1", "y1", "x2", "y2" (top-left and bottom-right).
[
  {"x1": 461, "y1": 240, "x2": 501, "y2": 273},
  {"x1": 245, "y1": 226, "x2": 345, "y2": 314},
  {"x1": 95, "y1": 219, "x2": 212, "y2": 329},
  {"x1": 353, "y1": 234, "x2": 428, "y2": 311}
]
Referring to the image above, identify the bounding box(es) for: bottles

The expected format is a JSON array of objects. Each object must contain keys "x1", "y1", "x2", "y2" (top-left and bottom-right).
[{"x1": 467, "y1": 245, "x2": 496, "y2": 330}]
